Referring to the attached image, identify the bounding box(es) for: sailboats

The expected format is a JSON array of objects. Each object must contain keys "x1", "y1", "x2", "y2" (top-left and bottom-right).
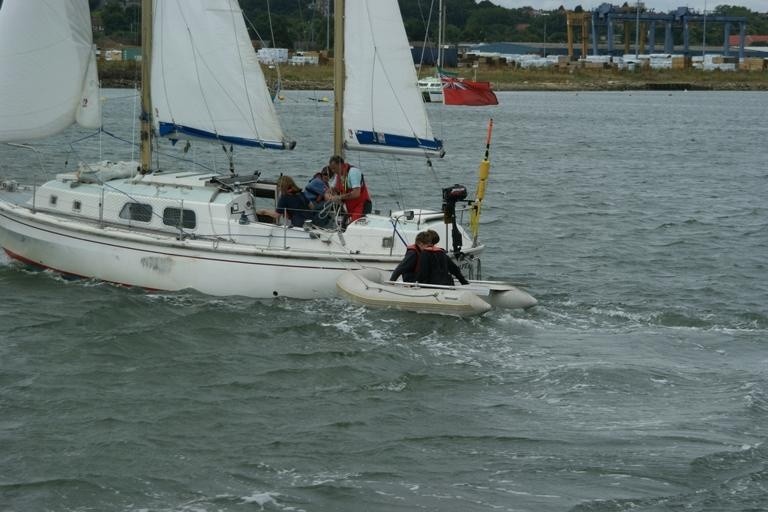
[
  {"x1": 1, "y1": 0, "x2": 495, "y2": 299},
  {"x1": 416, "y1": 0, "x2": 497, "y2": 107}
]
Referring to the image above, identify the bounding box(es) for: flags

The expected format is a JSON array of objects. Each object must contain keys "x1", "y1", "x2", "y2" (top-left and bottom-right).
[{"x1": 439, "y1": 73, "x2": 466, "y2": 91}]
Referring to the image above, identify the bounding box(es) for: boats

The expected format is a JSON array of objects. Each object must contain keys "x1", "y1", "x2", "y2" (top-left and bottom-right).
[{"x1": 337, "y1": 267, "x2": 540, "y2": 315}]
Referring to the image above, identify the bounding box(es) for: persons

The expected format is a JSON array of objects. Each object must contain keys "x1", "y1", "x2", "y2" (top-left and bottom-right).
[
  {"x1": 393, "y1": 231, "x2": 432, "y2": 287},
  {"x1": 304, "y1": 167, "x2": 335, "y2": 226},
  {"x1": 256, "y1": 176, "x2": 314, "y2": 228},
  {"x1": 328, "y1": 156, "x2": 372, "y2": 231},
  {"x1": 415, "y1": 229, "x2": 469, "y2": 289}
]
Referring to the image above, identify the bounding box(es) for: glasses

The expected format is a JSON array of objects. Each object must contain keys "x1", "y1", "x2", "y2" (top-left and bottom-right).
[{"x1": 323, "y1": 172, "x2": 332, "y2": 179}]
